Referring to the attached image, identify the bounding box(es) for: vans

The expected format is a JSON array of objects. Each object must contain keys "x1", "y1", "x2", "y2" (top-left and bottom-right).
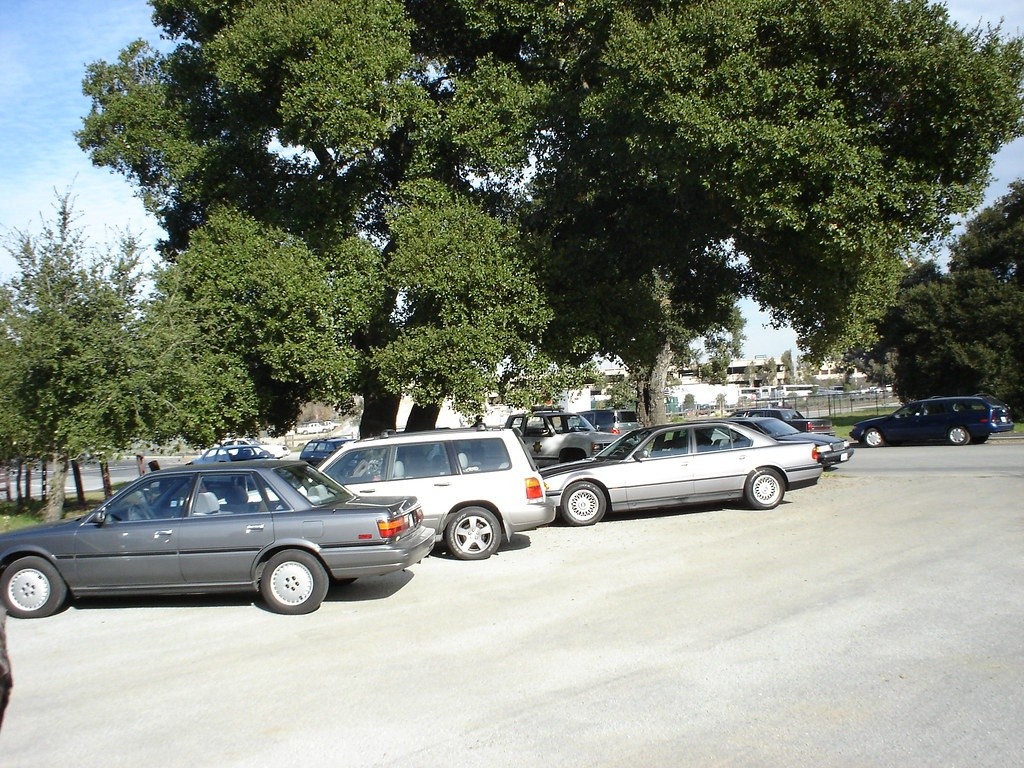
[{"x1": 577, "y1": 409, "x2": 638, "y2": 435}]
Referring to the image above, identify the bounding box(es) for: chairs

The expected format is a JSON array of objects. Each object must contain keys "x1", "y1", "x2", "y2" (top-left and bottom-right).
[
  {"x1": 661, "y1": 441, "x2": 674, "y2": 451},
  {"x1": 196, "y1": 492, "x2": 221, "y2": 515},
  {"x1": 307, "y1": 485, "x2": 328, "y2": 498},
  {"x1": 394, "y1": 460, "x2": 405, "y2": 479},
  {"x1": 458, "y1": 454, "x2": 468, "y2": 471},
  {"x1": 223, "y1": 487, "x2": 250, "y2": 512}
]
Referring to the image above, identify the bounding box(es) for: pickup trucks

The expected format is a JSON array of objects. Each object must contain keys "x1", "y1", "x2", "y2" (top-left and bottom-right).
[
  {"x1": 324, "y1": 421, "x2": 339, "y2": 430},
  {"x1": 503, "y1": 406, "x2": 633, "y2": 469},
  {"x1": 297, "y1": 423, "x2": 328, "y2": 434}
]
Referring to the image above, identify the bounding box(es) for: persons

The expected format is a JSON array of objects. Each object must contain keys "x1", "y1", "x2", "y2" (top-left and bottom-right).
[{"x1": 470, "y1": 415, "x2": 486, "y2": 428}]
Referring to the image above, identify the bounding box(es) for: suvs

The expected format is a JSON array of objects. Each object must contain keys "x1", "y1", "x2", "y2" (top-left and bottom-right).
[
  {"x1": 300, "y1": 437, "x2": 358, "y2": 467},
  {"x1": 848, "y1": 393, "x2": 1014, "y2": 448},
  {"x1": 217, "y1": 423, "x2": 557, "y2": 561}
]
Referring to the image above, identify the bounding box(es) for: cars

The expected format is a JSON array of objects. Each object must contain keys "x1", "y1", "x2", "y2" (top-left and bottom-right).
[
  {"x1": 186, "y1": 445, "x2": 276, "y2": 465},
  {"x1": 202, "y1": 439, "x2": 290, "y2": 459},
  {"x1": 695, "y1": 418, "x2": 854, "y2": 472},
  {"x1": 535, "y1": 421, "x2": 824, "y2": 527},
  {"x1": 0, "y1": 457, "x2": 437, "y2": 618},
  {"x1": 850, "y1": 386, "x2": 896, "y2": 400},
  {"x1": 724, "y1": 409, "x2": 836, "y2": 438}
]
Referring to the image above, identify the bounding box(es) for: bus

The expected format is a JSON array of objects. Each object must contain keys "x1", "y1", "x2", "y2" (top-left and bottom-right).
[
  {"x1": 775, "y1": 385, "x2": 819, "y2": 398},
  {"x1": 739, "y1": 386, "x2": 776, "y2": 400}
]
